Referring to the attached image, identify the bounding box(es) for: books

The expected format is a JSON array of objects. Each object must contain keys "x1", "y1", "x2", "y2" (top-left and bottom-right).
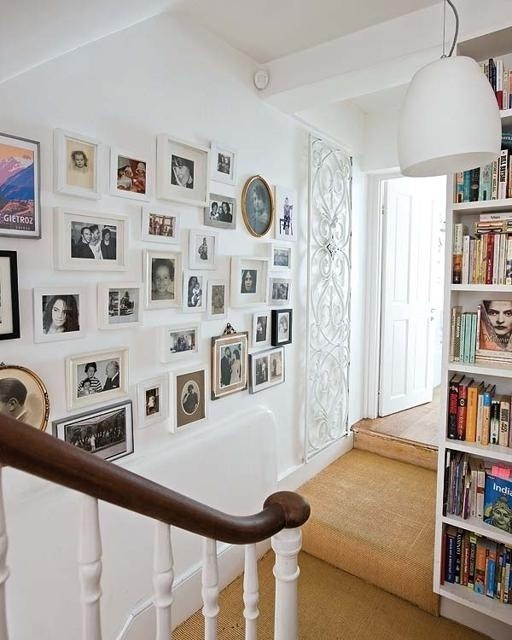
[{"x1": 442, "y1": 56, "x2": 512, "y2": 608}]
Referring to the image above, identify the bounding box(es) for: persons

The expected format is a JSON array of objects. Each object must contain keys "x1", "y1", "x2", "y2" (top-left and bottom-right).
[
  {"x1": 149, "y1": 216, "x2": 172, "y2": 235},
  {"x1": 78, "y1": 361, "x2": 119, "y2": 396},
  {"x1": 72, "y1": 425, "x2": 123, "y2": 451},
  {"x1": 109, "y1": 292, "x2": 130, "y2": 315},
  {"x1": 249, "y1": 185, "x2": 269, "y2": 233},
  {"x1": 68, "y1": 152, "x2": 90, "y2": 188},
  {"x1": 241, "y1": 269, "x2": 288, "y2": 341},
  {"x1": 221, "y1": 347, "x2": 278, "y2": 386},
  {"x1": 211, "y1": 153, "x2": 232, "y2": 223},
  {"x1": 188, "y1": 238, "x2": 223, "y2": 313},
  {"x1": 148, "y1": 391, "x2": 156, "y2": 412},
  {"x1": 173, "y1": 158, "x2": 191, "y2": 187},
  {"x1": 275, "y1": 198, "x2": 293, "y2": 265},
  {"x1": 183, "y1": 384, "x2": 198, "y2": 412},
  {"x1": 0, "y1": 378, "x2": 31, "y2": 422},
  {"x1": 152, "y1": 259, "x2": 175, "y2": 299},
  {"x1": 71, "y1": 225, "x2": 116, "y2": 259},
  {"x1": 171, "y1": 335, "x2": 189, "y2": 351},
  {"x1": 42, "y1": 295, "x2": 80, "y2": 333},
  {"x1": 117, "y1": 163, "x2": 146, "y2": 193}
]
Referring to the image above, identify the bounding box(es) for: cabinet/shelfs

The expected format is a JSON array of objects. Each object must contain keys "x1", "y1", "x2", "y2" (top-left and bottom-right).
[{"x1": 429, "y1": 25, "x2": 512, "y2": 639}]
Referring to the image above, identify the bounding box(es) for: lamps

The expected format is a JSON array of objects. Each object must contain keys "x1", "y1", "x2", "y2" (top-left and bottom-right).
[{"x1": 393, "y1": 1, "x2": 502, "y2": 186}]
